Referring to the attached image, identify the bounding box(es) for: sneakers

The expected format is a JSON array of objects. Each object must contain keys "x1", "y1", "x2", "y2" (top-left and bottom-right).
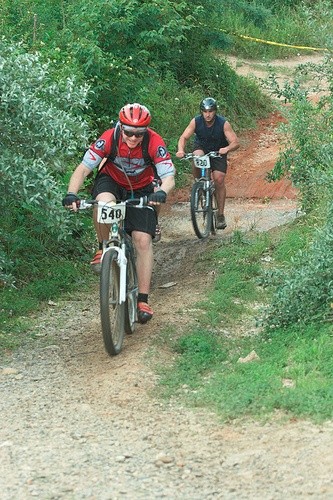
[
  {"x1": 138, "y1": 302, "x2": 154, "y2": 320},
  {"x1": 90, "y1": 250, "x2": 102, "y2": 265}
]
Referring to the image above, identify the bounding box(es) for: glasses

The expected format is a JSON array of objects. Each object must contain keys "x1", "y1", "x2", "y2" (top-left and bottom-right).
[{"x1": 124, "y1": 130, "x2": 147, "y2": 138}]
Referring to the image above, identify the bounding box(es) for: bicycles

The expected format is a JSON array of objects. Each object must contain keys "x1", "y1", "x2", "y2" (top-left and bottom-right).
[
  {"x1": 180, "y1": 150, "x2": 222, "y2": 238},
  {"x1": 68, "y1": 196, "x2": 166, "y2": 356}
]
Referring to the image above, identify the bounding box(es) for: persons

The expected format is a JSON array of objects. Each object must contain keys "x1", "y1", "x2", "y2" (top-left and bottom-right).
[
  {"x1": 62, "y1": 103, "x2": 175, "y2": 325},
  {"x1": 176, "y1": 97, "x2": 240, "y2": 229}
]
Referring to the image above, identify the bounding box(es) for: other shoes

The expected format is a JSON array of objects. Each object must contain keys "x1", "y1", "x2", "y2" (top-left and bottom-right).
[{"x1": 216, "y1": 215, "x2": 227, "y2": 229}]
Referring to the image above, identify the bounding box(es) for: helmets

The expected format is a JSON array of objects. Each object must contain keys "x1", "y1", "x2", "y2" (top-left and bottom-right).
[
  {"x1": 200, "y1": 98, "x2": 216, "y2": 111},
  {"x1": 119, "y1": 103, "x2": 151, "y2": 127}
]
